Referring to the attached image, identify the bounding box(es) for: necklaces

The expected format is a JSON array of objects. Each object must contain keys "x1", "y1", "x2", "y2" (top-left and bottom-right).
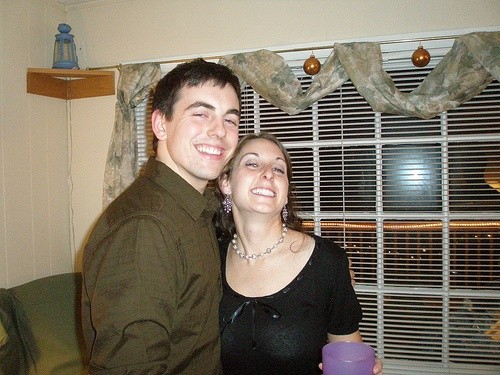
[{"x1": 231, "y1": 222, "x2": 288, "y2": 260}]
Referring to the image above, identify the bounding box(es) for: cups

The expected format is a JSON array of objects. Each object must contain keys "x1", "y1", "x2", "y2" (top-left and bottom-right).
[{"x1": 322, "y1": 339, "x2": 374, "y2": 375}]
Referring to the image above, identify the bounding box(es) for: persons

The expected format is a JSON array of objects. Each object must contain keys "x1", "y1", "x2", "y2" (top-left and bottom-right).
[
  {"x1": 79, "y1": 57, "x2": 357, "y2": 374},
  {"x1": 213, "y1": 133, "x2": 385, "y2": 375}
]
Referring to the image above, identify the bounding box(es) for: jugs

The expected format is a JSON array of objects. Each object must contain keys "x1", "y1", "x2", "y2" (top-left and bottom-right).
[{"x1": 51, "y1": 23, "x2": 79, "y2": 69}]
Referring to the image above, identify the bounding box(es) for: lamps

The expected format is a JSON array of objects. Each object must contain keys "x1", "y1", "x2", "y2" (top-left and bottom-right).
[{"x1": 51, "y1": 22, "x2": 80, "y2": 70}]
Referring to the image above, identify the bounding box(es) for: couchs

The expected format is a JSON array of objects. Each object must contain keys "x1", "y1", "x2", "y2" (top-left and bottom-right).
[{"x1": 0, "y1": 271, "x2": 89, "y2": 375}]
